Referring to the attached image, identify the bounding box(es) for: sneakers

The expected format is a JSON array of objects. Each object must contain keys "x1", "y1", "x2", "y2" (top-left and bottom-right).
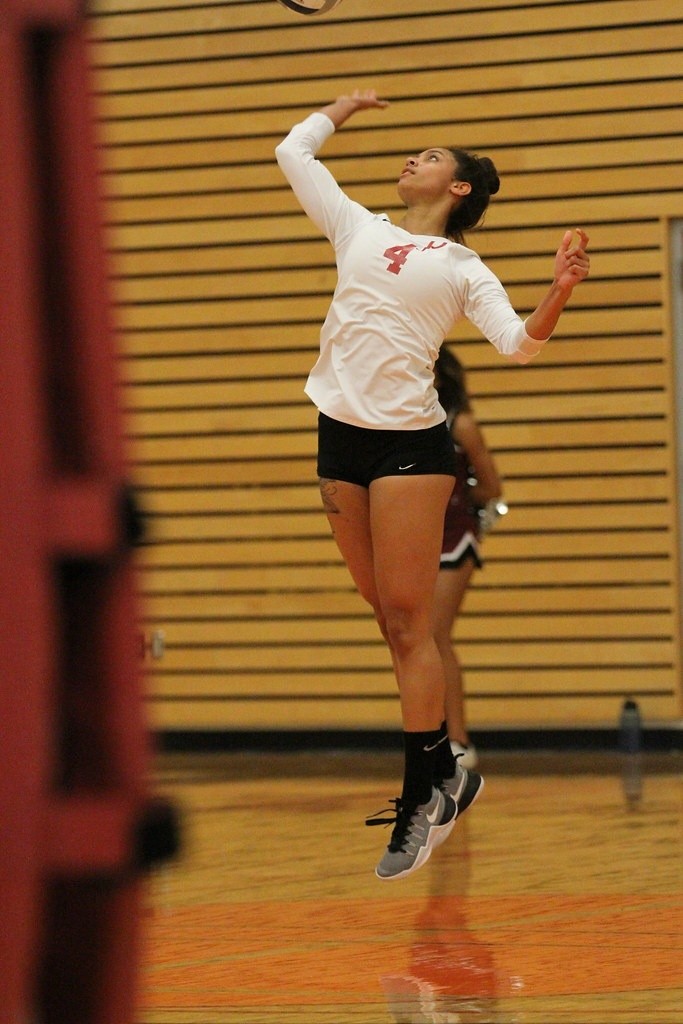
[
  {"x1": 450, "y1": 738, "x2": 478, "y2": 770},
  {"x1": 364, "y1": 764, "x2": 484, "y2": 880}
]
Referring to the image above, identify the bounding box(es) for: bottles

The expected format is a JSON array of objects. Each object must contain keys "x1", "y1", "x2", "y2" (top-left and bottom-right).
[{"x1": 620, "y1": 702, "x2": 640, "y2": 751}]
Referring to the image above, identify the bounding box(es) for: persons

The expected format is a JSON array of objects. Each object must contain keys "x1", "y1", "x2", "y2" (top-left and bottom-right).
[
  {"x1": 434, "y1": 341, "x2": 503, "y2": 767},
  {"x1": 273, "y1": 85, "x2": 590, "y2": 879}
]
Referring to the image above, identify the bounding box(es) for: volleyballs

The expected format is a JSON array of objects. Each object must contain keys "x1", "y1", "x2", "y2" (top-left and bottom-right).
[{"x1": 278, "y1": 0, "x2": 342, "y2": 16}]
